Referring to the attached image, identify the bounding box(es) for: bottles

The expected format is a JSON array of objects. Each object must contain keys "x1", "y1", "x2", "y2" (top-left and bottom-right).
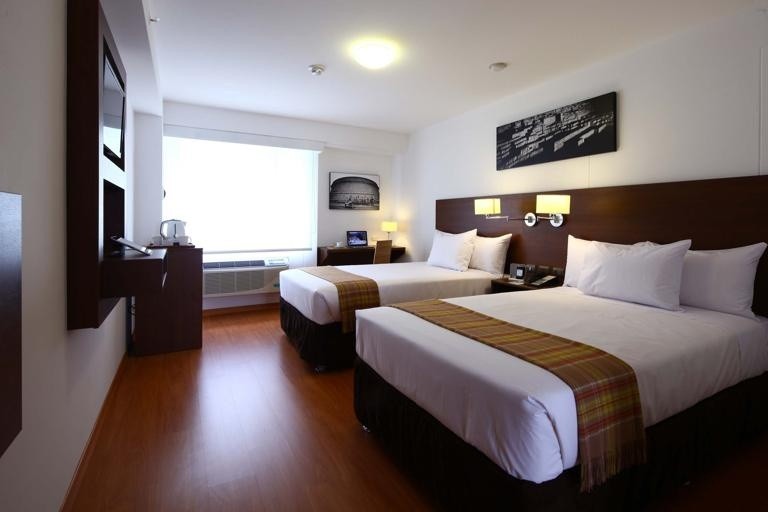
[{"x1": 516, "y1": 264, "x2": 525, "y2": 279}]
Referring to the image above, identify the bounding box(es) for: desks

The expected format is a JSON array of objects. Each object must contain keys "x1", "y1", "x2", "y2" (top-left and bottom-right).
[{"x1": 317, "y1": 245, "x2": 405, "y2": 266}]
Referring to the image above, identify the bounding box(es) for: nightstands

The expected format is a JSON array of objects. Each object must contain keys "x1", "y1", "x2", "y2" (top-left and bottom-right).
[{"x1": 491, "y1": 275, "x2": 561, "y2": 293}]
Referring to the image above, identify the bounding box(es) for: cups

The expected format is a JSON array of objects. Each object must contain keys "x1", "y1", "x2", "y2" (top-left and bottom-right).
[
  {"x1": 336, "y1": 242, "x2": 345, "y2": 246},
  {"x1": 152, "y1": 235, "x2": 191, "y2": 245}
]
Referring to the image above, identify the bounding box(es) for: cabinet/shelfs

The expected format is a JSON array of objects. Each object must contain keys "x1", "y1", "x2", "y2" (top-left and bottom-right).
[{"x1": 133, "y1": 247, "x2": 203, "y2": 357}]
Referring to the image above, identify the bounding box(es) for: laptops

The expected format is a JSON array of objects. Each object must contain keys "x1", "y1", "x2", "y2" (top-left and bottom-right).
[{"x1": 347, "y1": 230, "x2": 370, "y2": 248}]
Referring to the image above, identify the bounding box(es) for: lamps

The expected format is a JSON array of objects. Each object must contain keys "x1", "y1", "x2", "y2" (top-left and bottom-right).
[
  {"x1": 474, "y1": 197, "x2": 537, "y2": 227},
  {"x1": 535, "y1": 194, "x2": 571, "y2": 228},
  {"x1": 380, "y1": 221, "x2": 398, "y2": 240}
]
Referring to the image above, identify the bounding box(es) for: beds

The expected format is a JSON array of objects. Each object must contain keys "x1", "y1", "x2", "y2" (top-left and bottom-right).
[
  {"x1": 278, "y1": 260, "x2": 515, "y2": 374},
  {"x1": 354, "y1": 285, "x2": 768, "y2": 512}
]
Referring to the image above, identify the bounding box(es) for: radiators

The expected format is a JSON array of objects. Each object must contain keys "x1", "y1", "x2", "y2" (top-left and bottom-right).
[{"x1": 202, "y1": 266, "x2": 290, "y2": 299}]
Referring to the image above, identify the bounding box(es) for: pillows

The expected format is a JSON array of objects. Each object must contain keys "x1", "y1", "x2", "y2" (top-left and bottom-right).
[
  {"x1": 646, "y1": 239, "x2": 768, "y2": 323},
  {"x1": 427, "y1": 228, "x2": 478, "y2": 271},
  {"x1": 468, "y1": 233, "x2": 513, "y2": 277},
  {"x1": 579, "y1": 238, "x2": 692, "y2": 312},
  {"x1": 561, "y1": 233, "x2": 585, "y2": 296}
]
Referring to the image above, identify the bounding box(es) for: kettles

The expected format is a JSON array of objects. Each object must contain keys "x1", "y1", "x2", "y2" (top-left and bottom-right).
[{"x1": 160, "y1": 219, "x2": 186, "y2": 239}]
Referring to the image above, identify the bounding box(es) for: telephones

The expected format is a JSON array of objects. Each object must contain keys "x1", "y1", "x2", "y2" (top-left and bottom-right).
[{"x1": 525, "y1": 271, "x2": 559, "y2": 287}]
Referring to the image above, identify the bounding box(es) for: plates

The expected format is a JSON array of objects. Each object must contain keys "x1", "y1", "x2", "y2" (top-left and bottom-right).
[{"x1": 335, "y1": 245, "x2": 345, "y2": 248}]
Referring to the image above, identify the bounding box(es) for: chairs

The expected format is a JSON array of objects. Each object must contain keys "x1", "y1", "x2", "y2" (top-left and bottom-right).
[{"x1": 372, "y1": 240, "x2": 392, "y2": 263}]
